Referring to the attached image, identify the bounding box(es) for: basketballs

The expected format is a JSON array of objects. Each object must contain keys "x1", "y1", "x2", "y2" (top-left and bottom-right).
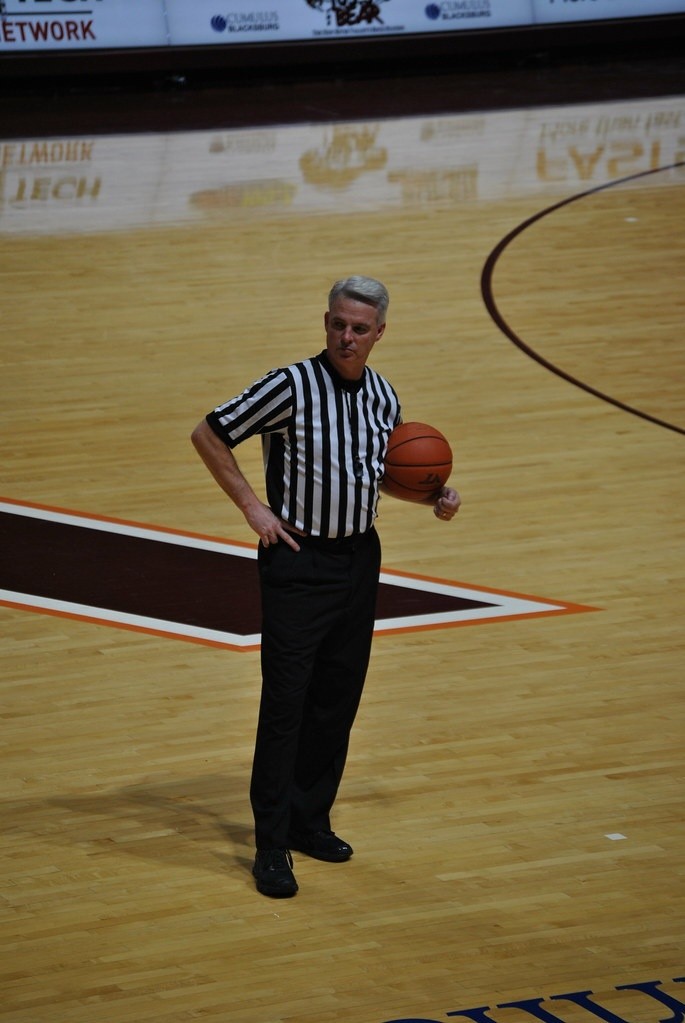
[{"x1": 378, "y1": 421, "x2": 454, "y2": 503}]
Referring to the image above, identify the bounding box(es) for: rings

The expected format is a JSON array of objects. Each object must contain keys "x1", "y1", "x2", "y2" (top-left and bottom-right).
[{"x1": 443, "y1": 513, "x2": 446, "y2": 517}]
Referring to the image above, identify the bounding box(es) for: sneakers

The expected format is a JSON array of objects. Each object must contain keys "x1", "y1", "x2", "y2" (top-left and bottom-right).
[
  {"x1": 252, "y1": 845, "x2": 299, "y2": 896},
  {"x1": 292, "y1": 829, "x2": 354, "y2": 861}
]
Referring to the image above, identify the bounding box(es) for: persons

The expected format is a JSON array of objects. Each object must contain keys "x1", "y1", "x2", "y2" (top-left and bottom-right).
[{"x1": 192, "y1": 275, "x2": 462, "y2": 897}]
